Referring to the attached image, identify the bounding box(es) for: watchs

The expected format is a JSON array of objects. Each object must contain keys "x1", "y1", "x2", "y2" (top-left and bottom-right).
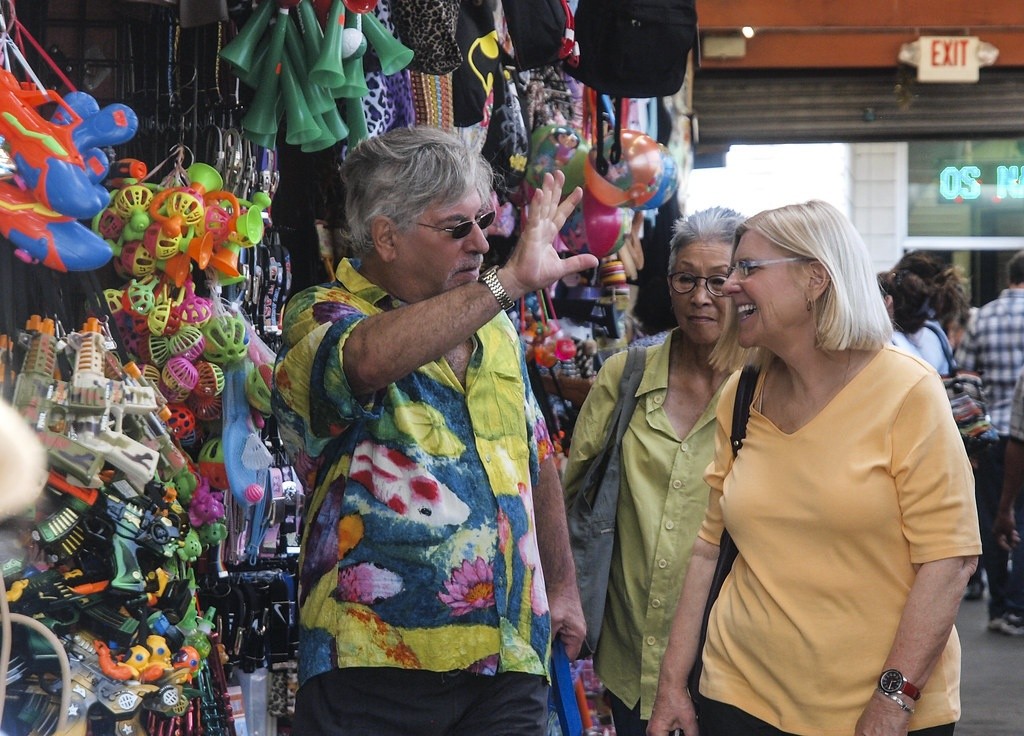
[
  {"x1": 477, "y1": 264, "x2": 516, "y2": 313},
  {"x1": 878, "y1": 669, "x2": 920, "y2": 702}
]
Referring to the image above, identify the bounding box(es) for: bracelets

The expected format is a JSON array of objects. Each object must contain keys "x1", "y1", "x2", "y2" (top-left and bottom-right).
[{"x1": 875, "y1": 687, "x2": 915, "y2": 715}]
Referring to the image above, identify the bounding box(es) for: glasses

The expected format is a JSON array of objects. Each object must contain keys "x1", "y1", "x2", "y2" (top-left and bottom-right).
[
  {"x1": 668, "y1": 272, "x2": 735, "y2": 297},
  {"x1": 387, "y1": 208, "x2": 496, "y2": 239},
  {"x1": 728, "y1": 255, "x2": 812, "y2": 283}
]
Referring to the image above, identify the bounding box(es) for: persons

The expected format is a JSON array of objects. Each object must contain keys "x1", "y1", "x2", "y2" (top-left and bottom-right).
[
  {"x1": 876, "y1": 248, "x2": 1023, "y2": 635},
  {"x1": 562, "y1": 206, "x2": 748, "y2": 736},
  {"x1": 269, "y1": 125, "x2": 598, "y2": 736},
  {"x1": 685, "y1": 200, "x2": 983, "y2": 736}
]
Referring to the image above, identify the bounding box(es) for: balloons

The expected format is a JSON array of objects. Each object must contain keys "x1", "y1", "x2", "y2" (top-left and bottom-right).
[{"x1": 525, "y1": 125, "x2": 680, "y2": 258}]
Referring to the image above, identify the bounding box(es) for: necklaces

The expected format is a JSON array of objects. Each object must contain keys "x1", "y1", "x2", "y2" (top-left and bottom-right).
[{"x1": 844, "y1": 348, "x2": 852, "y2": 387}]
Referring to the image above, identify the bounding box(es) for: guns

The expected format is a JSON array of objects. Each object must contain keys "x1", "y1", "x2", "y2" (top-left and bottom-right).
[{"x1": 0, "y1": 61, "x2": 239, "y2": 736}]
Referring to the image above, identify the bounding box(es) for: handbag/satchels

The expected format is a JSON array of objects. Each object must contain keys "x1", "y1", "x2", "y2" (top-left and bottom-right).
[
  {"x1": 559, "y1": 346, "x2": 647, "y2": 657},
  {"x1": 922, "y1": 322, "x2": 1000, "y2": 453}
]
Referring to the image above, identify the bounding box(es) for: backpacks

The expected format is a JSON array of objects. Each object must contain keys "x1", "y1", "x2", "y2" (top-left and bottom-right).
[{"x1": 560, "y1": 0, "x2": 698, "y2": 98}]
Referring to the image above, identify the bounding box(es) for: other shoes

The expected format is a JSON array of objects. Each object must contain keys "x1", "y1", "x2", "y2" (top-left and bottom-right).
[
  {"x1": 989, "y1": 610, "x2": 1024, "y2": 636},
  {"x1": 963, "y1": 583, "x2": 982, "y2": 599}
]
